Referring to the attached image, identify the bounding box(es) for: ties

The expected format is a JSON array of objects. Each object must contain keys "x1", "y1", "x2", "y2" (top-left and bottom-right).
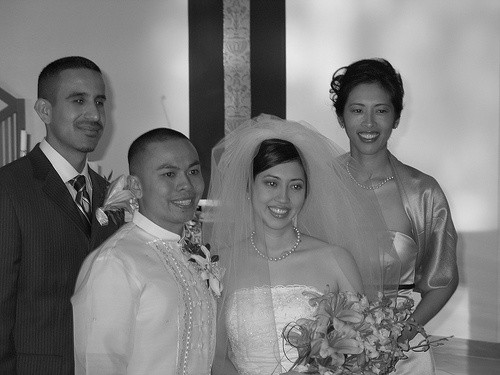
[{"x1": 66, "y1": 175, "x2": 92, "y2": 222}]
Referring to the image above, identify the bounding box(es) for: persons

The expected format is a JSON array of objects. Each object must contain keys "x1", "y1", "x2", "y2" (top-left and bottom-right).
[
  {"x1": 70, "y1": 128, "x2": 218, "y2": 375},
  {"x1": 202, "y1": 114, "x2": 399, "y2": 375},
  {"x1": 0, "y1": 56, "x2": 135, "y2": 375},
  {"x1": 330, "y1": 58, "x2": 459, "y2": 375}
]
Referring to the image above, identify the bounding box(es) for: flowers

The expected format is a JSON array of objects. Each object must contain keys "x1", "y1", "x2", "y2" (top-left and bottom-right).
[
  {"x1": 95, "y1": 169, "x2": 140, "y2": 227},
  {"x1": 182, "y1": 240, "x2": 226, "y2": 299},
  {"x1": 281, "y1": 284, "x2": 453, "y2": 375}
]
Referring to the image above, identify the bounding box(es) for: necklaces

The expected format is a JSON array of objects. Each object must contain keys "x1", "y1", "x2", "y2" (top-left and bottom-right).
[
  {"x1": 346, "y1": 159, "x2": 394, "y2": 190},
  {"x1": 250, "y1": 225, "x2": 301, "y2": 261}
]
